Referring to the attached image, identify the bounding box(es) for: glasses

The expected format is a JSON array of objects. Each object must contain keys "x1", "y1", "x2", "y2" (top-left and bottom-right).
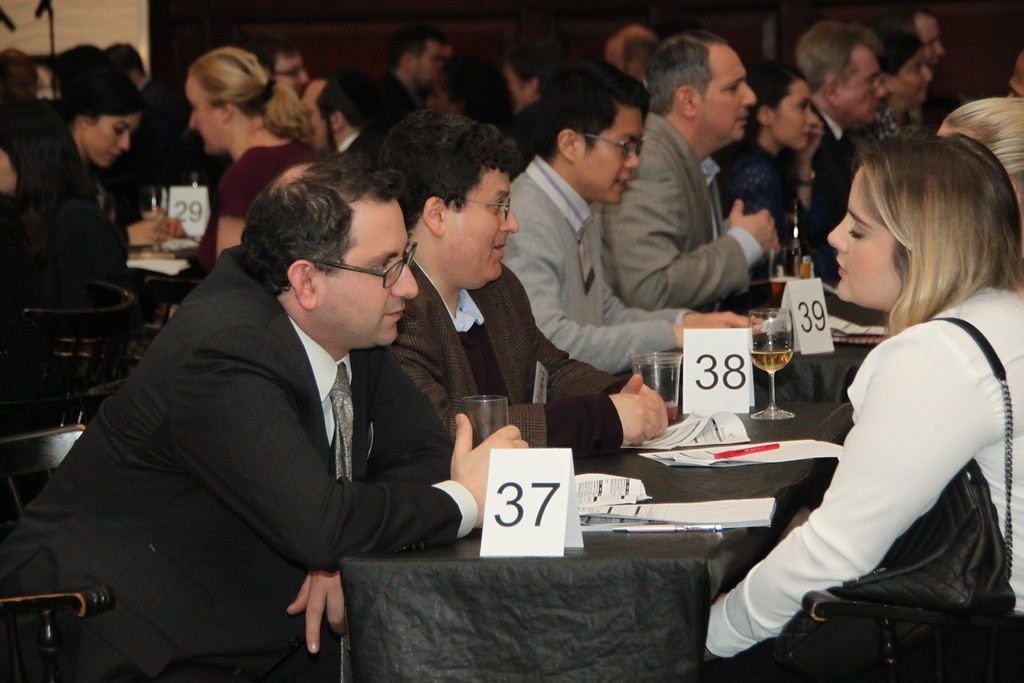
[
  {"x1": 831, "y1": 68, "x2": 889, "y2": 97},
  {"x1": 444, "y1": 194, "x2": 511, "y2": 222},
  {"x1": 579, "y1": 130, "x2": 643, "y2": 160},
  {"x1": 271, "y1": 60, "x2": 312, "y2": 82},
  {"x1": 284, "y1": 239, "x2": 419, "y2": 290}
]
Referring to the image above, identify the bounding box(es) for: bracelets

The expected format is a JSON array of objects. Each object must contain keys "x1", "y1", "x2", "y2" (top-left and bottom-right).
[{"x1": 798, "y1": 179, "x2": 813, "y2": 186}]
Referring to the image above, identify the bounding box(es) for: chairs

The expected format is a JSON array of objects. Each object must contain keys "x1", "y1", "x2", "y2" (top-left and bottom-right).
[{"x1": 0, "y1": 261, "x2": 205, "y2": 683}]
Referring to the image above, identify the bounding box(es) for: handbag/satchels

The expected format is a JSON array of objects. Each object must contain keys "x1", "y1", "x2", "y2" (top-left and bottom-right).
[{"x1": 771, "y1": 317, "x2": 1024, "y2": 683}]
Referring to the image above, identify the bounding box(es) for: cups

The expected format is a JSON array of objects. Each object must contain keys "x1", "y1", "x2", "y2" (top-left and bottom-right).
[
  {"x1": 461, "y1": 395, "x2": 508, "y2": 450},
  {"x1": 628, "y1": 352, "x2": 686, "y2": 421}
]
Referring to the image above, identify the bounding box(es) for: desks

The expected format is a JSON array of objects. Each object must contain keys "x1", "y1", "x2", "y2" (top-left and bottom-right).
[{"x1": 348, "y1": 283, "x2": 897, "y2": 683}]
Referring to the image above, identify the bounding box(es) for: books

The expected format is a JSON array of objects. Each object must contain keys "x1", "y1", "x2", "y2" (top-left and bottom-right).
[
  {"x1": 834, "y1": 334, "x2": 883, "y2": 344},
  {"x1": 0, "y1": 0, "x2": 1024, "y2": 683}
]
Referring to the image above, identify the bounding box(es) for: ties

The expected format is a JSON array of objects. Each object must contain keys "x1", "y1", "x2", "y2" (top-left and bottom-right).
[
  {"x1": 574, "y1": 226, "x2": 596, "y2": 295},
  {"x1": 328, "y1": 362, "x2": 355, "y2": 482}
]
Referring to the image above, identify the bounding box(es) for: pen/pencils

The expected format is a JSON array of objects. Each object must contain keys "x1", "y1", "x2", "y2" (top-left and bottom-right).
[
  {"x1": 714, "y1": 443, "x2": 780, "y2": 459},
  {"x1": 613, "y1": 525, "x2": 722, "y2": 533},
  {"x1": 141, "y1": 252, "x2": 175, "y2": 259}
]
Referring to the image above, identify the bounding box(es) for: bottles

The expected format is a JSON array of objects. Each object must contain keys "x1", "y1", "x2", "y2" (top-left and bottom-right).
[
  {"x1": 774, "y1": 198, "x2": 802, "y2": 276},
  {"x1": 799, "y1": 255, "x2": 814, "y2": 279}
]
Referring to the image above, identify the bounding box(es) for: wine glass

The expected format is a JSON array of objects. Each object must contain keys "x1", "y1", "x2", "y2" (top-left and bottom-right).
[
  {"x1": 138, "y1": 185, "x2": 168, "y2": 251},
  {"x1": 748, "y1": 307, "x2": 795, "y2": 420}
]
